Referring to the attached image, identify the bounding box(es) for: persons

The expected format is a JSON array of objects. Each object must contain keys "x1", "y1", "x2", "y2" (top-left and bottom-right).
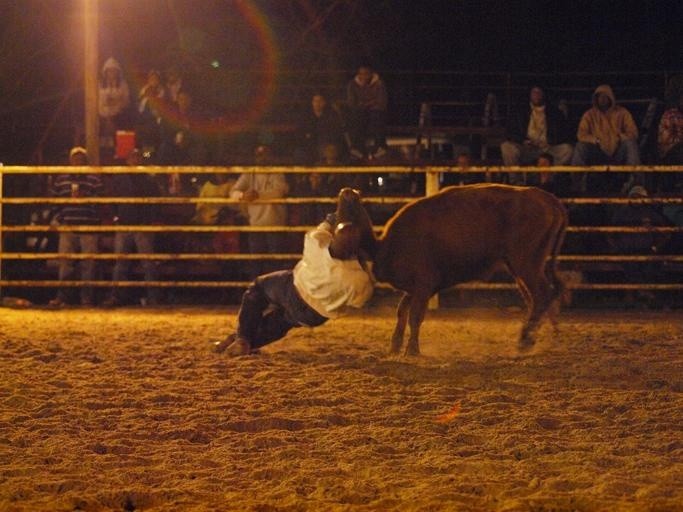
[
  {"x1": 216, "y1": 213, "x2": 374, "y2": 357},
  {"x1": 47, "y1": 57, "x2": 210, "y2": 308},
  {"x1": 180, "y1": 141, "x2": 292, "y2": 306},
  {"x1": 442, "y1": 84, "x2": 682, "y2": 298},
  {"x1": 289, "y1": 61, "x2": 403, "y2": 239}
]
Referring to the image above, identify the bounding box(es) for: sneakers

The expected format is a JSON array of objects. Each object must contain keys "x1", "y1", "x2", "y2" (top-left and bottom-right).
[{"x1": 210, "y1": 333, "x2": 252, "y2": 358}]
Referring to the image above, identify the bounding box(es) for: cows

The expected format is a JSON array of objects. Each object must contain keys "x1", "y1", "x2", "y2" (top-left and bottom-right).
[{"x1": 327, "y1": 179, "x2": 570, "y2": 356}]
